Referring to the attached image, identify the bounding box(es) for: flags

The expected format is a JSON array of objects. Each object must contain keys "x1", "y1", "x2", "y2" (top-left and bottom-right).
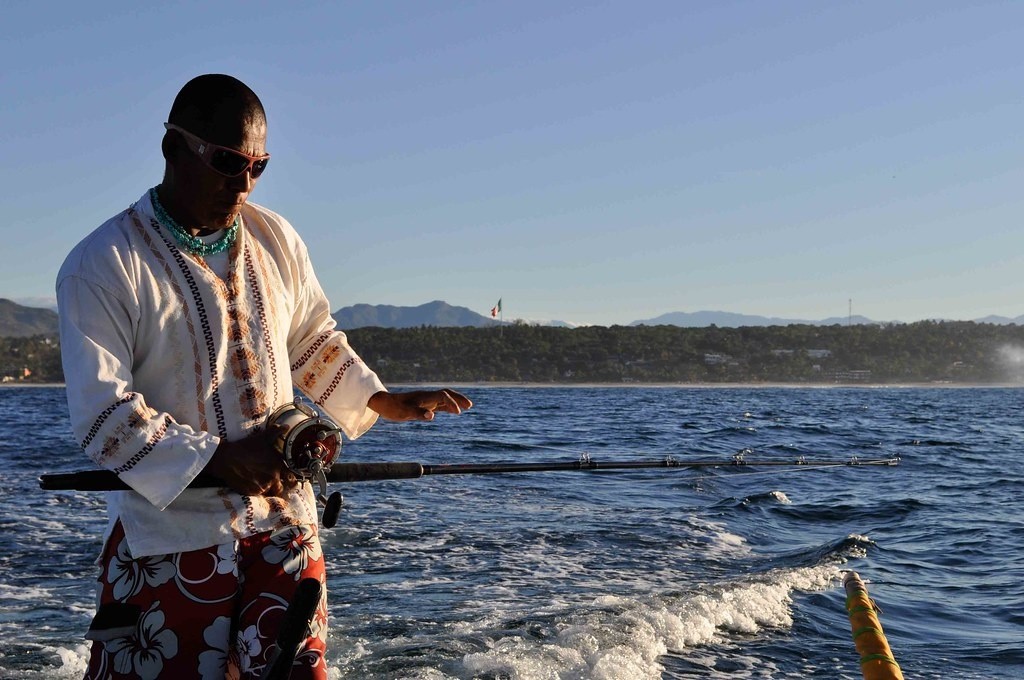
[{"x1": 491, "y1": 300, "x2": 502, "y2": 316}]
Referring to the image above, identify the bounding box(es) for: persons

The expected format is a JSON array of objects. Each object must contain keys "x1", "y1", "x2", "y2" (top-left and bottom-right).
[{"x1": 54, "y1": 74, "x2": 473, "y2": 680}]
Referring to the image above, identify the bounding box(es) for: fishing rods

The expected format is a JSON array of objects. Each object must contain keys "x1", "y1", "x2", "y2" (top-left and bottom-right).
[{"x1": 35, "y1": 393, "x2": 902, "y2": 497}]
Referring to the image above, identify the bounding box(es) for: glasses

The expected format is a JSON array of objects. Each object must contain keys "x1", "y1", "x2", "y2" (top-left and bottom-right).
[{"x1": 163, "y1": 120, "x2": 271, "y2": 179}]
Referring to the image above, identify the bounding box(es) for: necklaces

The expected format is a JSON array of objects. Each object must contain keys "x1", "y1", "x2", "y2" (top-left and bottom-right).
[{"x1": 153, "y1": 185, "x2": 239, "y2": 256}]
